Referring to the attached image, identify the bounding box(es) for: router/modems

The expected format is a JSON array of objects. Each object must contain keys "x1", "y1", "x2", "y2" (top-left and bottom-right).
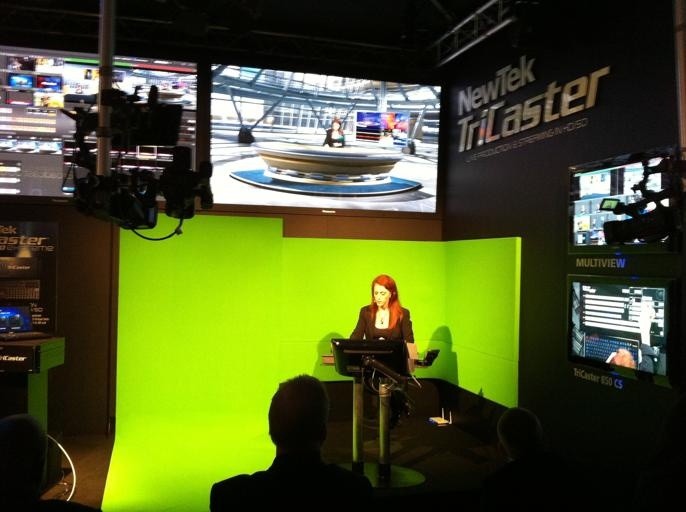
[{"x1": 428, "y1": 407, "x2": 453, "y2": 427}]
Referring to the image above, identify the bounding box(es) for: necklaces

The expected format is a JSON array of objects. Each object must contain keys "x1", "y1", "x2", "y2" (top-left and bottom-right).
[{"x1": 377, "y1": 310, "x2": 388, "y2": 325}]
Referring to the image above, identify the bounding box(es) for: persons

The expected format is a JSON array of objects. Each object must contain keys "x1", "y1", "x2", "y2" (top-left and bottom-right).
[
  {"x1": 322, "y1": 118, "x2": 345, "y2": 147},
  {"x1": 352, "y1": 275, "x2": 418, "y2": 373},
  {"x1": 0, "y1": 413, "x2": 101, "y2": 512},
  {"x1": 632, "y1": 329, "x2": 686, "y2": 511},
  {"x1": 480, "y1": 406, "x2": 589, "y2": 510},
  {"x1": 210, "y1": 374, "x2": 379, "y2": 511}
]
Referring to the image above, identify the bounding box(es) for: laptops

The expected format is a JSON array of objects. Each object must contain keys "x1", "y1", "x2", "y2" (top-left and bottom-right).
[{"x1": 0, "y1": 303, "x2": 53, "y2": 341}]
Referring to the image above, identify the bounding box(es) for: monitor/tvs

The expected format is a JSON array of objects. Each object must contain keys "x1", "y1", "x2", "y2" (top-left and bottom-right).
[
  {"x1": 566, "y1": 142, "x2": 680, "y2": 255},
  {"x1": 1, "y1": 44, "x2": 202, "y2": 205},
  {"x1": 565, "y1": 272, "x2": 675, "y2": 390},
  {"x1": 330, "y1": 337, "x2": 409, "y2": 377},
  {"x1": 207, "y1": 62, "x2": 448, "y2": 220}
]
